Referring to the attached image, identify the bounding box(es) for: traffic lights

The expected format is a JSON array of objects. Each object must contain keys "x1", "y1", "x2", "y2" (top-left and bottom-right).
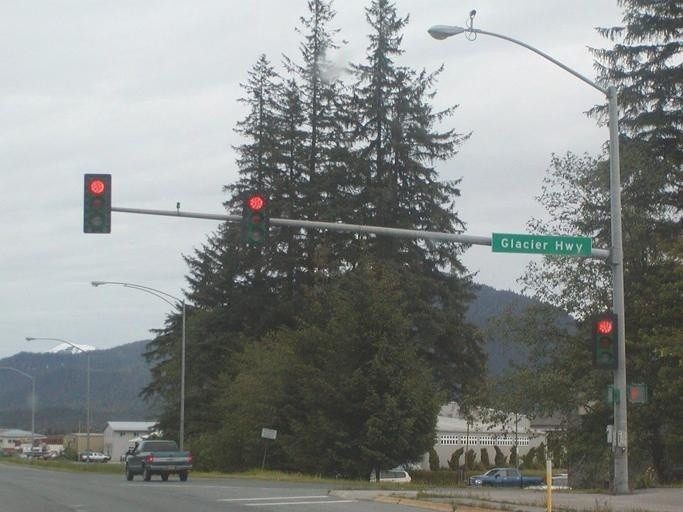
[
  {"x1": 593, "y1": 314, "x2": 617, "y2": 369},
  {"x1": 85, "y1": 174, "x2": 111, "y2": 234},
  {"x1": 242, "y1": 193, "x2": 268, "y2": 246}
]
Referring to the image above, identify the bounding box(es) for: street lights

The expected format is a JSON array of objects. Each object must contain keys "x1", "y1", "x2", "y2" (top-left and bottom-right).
[
  {"x1": 428, "y1": 24, "x2": 629, "y2": 495},
  {"x1": 27, "y1": 338, "x2": 90, "y2": 452},
  {"x1": 92, "y1": 281, "x2": 186, "y2": 451}
]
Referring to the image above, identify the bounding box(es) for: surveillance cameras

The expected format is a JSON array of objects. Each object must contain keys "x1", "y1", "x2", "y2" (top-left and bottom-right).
[{"x1": 469, "y1": 10, "x2": 477, "y2": 18}]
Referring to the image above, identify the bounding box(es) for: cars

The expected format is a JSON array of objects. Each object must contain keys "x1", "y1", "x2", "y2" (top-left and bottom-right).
[
  {"x1": 81, "y1": 452, "x2": 111, "y2": 463},
  {"x1": 370, "y1": 471, "x2": 411, "y2": 483},
  {"x1": 470, "y1": 468, "x2": 569, "y2": 489}
]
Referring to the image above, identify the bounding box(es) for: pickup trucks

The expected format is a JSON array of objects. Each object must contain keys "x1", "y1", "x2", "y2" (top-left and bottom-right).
[{"x1": 126, "y1": 439, "x2": 192, "y2": 481}]
